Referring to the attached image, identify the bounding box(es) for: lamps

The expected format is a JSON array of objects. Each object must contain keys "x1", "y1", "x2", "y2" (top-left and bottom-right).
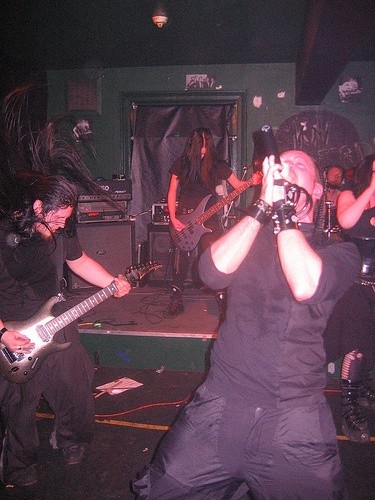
[{"x1": 152, "y1": 7, "x2": 170, "y2": 28}]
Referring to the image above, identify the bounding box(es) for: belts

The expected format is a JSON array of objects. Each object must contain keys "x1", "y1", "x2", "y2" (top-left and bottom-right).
[{"x1": 354, "y1": 278, "x2": 375, "y2": 286}]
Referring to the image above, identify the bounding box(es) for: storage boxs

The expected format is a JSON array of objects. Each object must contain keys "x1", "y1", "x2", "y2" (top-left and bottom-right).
[{"x1": 152, "y1": 204, "x2": 227, "y2": 226}]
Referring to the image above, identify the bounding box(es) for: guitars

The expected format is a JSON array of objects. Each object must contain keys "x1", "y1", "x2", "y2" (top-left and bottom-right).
[
  {"x1": 168, "y1": 170, "x2": 264, "y2": 252},
  {"x1": 0, "y1": 259, "x2": 163, "y2": 384}
]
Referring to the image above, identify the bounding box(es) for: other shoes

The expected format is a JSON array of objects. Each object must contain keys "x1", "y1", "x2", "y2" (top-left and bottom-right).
[
  {"x1": 14, "y1": 474, "x2": 39, "y2": 487},
  {"x1": 60, "y1": 447, "x2": 82, "y2": 465}
]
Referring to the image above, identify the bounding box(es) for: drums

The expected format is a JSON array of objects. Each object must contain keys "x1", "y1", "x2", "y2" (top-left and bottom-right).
[{"x1": 348, "y1": 234, "x2": 375, "y2": 288}]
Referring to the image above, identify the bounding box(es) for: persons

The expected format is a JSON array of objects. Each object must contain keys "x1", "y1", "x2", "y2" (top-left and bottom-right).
[
  {"x1": 128, "y1": 148, "x2": 362, "y2": 500},
  {"x1": 0, "y1": 85, "x2": 131, "y2": 487},
  {"x1": 162, "y1": 126, "x2": 264, "y2": 318},
  {"x1": 324, "y1": 154, "x2": 375, "y2": 444}
]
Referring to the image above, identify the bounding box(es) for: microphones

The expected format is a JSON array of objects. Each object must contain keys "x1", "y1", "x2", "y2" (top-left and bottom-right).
[{"x1": 261, "y1": 124, "x2": 284, "y2": 173}]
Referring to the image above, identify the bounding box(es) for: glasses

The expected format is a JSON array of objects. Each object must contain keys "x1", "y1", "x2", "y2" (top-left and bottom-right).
[{"x1": 328, "y1": 172, "x2": 343, "y2": 178}]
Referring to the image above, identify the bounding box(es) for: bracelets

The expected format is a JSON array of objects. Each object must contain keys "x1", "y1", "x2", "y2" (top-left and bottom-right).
[
  {"x1": 272, "y1": 210, "x2": 299, "y2": 236},
  {"x1": 249, "y1": 197, "x2": 275, "y2": 227}
]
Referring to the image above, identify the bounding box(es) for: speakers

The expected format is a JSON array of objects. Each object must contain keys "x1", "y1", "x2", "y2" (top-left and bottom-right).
[
  {"x1": 147, "y1": 223, "x2": 203, "y2": 287},
  {"x1": 67, "y1": 219, "x2": 137, "y2": 291}
]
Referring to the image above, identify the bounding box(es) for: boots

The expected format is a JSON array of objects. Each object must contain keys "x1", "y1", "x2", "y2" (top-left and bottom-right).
[
  {"x1": 341, "y1": 379, "x2": 367, "y2": 431},
  {"x1": 213, "y1": 292, "x2": 227, "y2": 340},
  {"x1": 169, "y1": 285, "x2": 184, "y2": 314},
  {"x1": 360, "y1": 369, "x2": 375, "y2": 401}
]
